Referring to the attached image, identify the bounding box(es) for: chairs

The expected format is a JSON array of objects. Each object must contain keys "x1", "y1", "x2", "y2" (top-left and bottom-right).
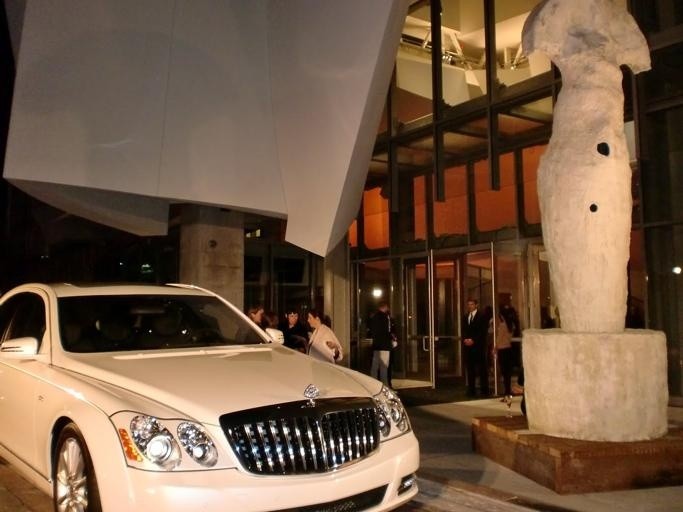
[{"x1": 71, "y1": 312, "x2": 146, "y2": 350}]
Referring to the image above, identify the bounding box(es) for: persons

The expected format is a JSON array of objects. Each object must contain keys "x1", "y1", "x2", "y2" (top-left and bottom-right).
[
  {"x1": 235, "y1": 300, "x2": 396, "y2": 386},
  {"x1": 462, "y1": 298, "x2": 556, "y2": 398}
]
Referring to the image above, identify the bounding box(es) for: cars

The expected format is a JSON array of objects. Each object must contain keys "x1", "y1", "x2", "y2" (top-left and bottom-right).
[{"x1": 0, "y1": 282, "x2": 419, "y2": 512}]
